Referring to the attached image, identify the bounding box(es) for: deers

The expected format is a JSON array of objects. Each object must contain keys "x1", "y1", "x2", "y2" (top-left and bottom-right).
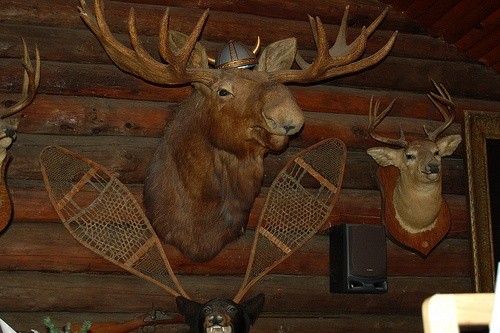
[
  {"x1": 76, "y1": 0, "x2": 409, "y2": 259},
  {"x1": 365, "y1": 77, "x2": 469, "y2": 231},
  {"x1": 0, "y1": 38, "x2": 44, "y2": 226}
]
